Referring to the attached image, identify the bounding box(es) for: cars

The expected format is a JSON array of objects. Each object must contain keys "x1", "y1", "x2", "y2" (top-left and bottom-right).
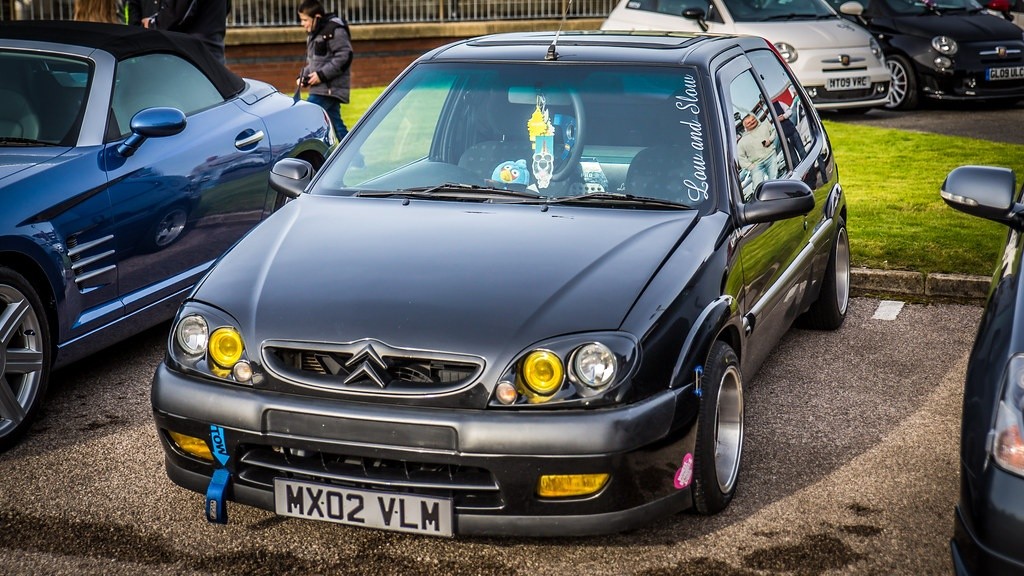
[
  {"x1": 596, "y1": 0, "x2": 894, "y2": 115},
  {"x1": 149, "y1": 27, "x2": 853, "y2": 544},
  {"x1": 762, "y1": 0, "x2": 1024, "y2": 113},
  {"x1": 0, "y1": 15, "x2": 346, "y2": 456},
  {"x1": 938, "y1": 164, "x2": 1024, "y2": 576}
]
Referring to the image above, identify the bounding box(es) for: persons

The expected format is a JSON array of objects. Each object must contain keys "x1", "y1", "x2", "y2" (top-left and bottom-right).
[
  {"x1": 759, "y1": 92, "x2": 807, "y2": 167},
  {"x1": 296, "y1": 0, "x2": 366, "y2": 170},
  {"x1": 739, "y1": 116, "x2": 776, "y2": 193},
  {"x1": 74, "y1": 0, "x2": 232, "y2": 67}
]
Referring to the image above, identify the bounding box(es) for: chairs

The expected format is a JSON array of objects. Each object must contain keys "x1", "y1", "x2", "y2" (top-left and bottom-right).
[
  {"x1": 626, "y1": 92, "x2": 705, "y2": 203},
  {"x1": 37, "y1": 87, "x2": 122, "y2": 146},
  {"x1": 453, "y1": 88, "x2": 539, "y2": 186}
]
